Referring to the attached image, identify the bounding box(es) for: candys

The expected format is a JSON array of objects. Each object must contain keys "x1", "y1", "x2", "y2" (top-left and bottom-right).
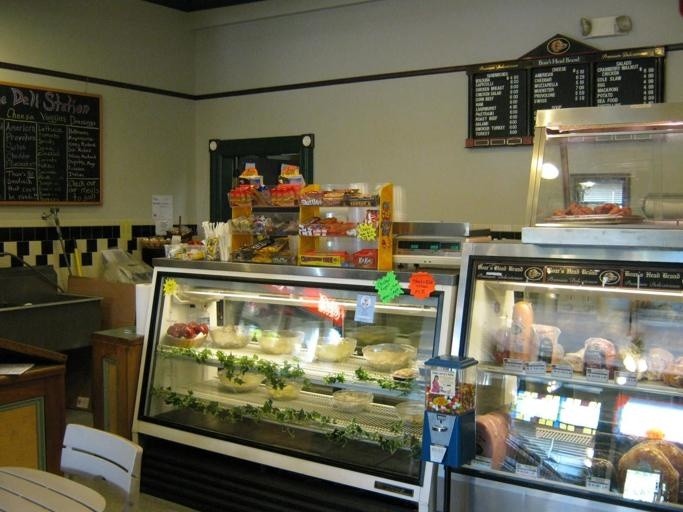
[{"x1": 425, "y1": 381, "x2": 475, "y2": 415}]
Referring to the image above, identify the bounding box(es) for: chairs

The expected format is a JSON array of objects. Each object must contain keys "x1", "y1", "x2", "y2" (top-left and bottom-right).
[{"x1": 59, "y1": 423, "x2": 143, "y2": 511}]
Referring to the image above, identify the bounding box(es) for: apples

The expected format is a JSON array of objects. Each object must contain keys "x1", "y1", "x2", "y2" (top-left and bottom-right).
[{"x1": 167, "y1": 321, "x2": 208, "y2": 339}]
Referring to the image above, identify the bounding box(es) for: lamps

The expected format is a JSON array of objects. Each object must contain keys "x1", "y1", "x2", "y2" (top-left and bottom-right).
[{"x1": 579, "y1": 14, "x2": 633, "y2": 39}]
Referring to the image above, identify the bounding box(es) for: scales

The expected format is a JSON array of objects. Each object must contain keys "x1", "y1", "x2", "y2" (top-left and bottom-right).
[{"x1": 392, "y1": 222, "x2": 493, "y2": 269}]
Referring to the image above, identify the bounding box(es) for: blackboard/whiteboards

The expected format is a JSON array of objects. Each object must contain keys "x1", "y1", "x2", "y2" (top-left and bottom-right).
[{"x1": 0, "y1": 80, "x2": 105, "y2": 206}]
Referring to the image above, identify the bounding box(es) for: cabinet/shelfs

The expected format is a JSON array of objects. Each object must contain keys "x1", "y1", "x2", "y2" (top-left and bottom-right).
[
  {"x1": 131, "y1": 223, "x2": 683, "y2": 512},
  {"x1": 522, "y1": 104, "x2": 682, "y2": 248},
  {"x1": 230, "y1": 183, "x2": 395, "y2": 271}
]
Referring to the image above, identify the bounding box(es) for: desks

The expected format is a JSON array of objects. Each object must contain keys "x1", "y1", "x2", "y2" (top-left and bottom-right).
[{"x1": 0, "y1": 466, "x2": 106, "y2": 512}]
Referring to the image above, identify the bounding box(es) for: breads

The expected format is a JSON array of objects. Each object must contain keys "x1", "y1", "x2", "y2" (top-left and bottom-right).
[{"x1": 475, "y1": 302, "x2": 683, "y2": 505}]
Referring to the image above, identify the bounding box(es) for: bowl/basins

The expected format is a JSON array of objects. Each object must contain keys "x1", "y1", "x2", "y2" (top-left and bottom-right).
[
  {"x1": 332, "y1": 390, "x2": 374, "y2": 413},
  {"x1": 209, "y1": 324, "x2": 257, "y2": 348},
  {"x1": 395, "y1": 400, "x2": 424, "y2": 428},
  {"x1": 260, "y1": 329, "x2": 305, "y2": 354},
  {"x1": 218, "y1": 375, "x2": 267, "y2": 393},
  {"x1": 265, "y1": 381, "x2": 303, "y2": 401},
  {"x1": 361, "y1": 342, "x2": 417, "y2": 371},
  {"x1": 312, "y1": 336, "x2": 358, "y2": 363}
]
